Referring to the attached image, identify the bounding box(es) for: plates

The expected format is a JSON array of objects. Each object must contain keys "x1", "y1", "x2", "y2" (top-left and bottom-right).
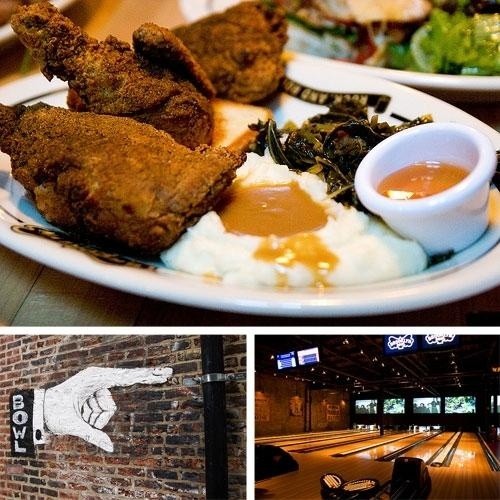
[
  {"x1": 1, "y1": 0, "x2": 74, "y2": 47},
  {"x1": 180, "y1": 1, "x2": 500, "y2": 108},
  {"x1": 0, "y1": 54, "x2": 500, "y2": 316}
]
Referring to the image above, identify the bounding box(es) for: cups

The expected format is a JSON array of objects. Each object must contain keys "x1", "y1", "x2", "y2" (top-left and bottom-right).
[{"x1": 355, "y1": 123, "x2": 498, "y2": 257}]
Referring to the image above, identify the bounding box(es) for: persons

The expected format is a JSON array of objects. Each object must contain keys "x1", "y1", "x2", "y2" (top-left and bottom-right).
[
  {"x1": 356, "y1": 398, "x2": 465, "y2": 414},
  {"x1": 10, "y1": 366, "x2": 175, "y2": 459}
]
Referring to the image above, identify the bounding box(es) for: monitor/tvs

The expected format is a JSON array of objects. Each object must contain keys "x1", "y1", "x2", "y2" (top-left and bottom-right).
[
  {"x1": 295, "y1": 345, "x2": 322, "y2": 368},
  {"x1": 275, "y1": 349, "x2": 297, "y2": 372},
  {"x1": 420, "y1": 335, "x2": 460, "y2": 352},
  {"x1": 383, "y1": 335, "x2": 420, "y2": 357}
]
